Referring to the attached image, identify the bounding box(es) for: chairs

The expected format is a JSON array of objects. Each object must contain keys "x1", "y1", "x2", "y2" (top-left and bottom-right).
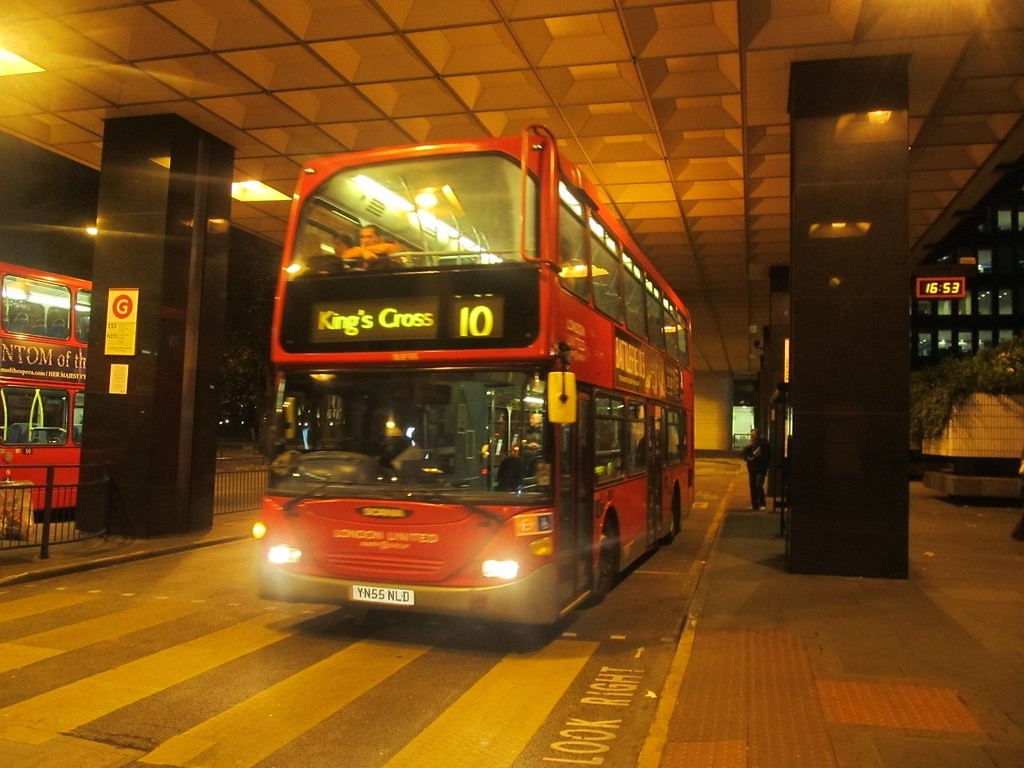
[
  {"x1": 7, "y1": 423, "x2": 41, "y2": 442},
  {"x1": 29, "y1": 319, "x2": 48, "y2": 335},
  {"x1": 50, "y1": 318, "x2": 67, "y2": 339},
  {"x1": 11, "y1": 312, "x2": 29, "y2": 332}
]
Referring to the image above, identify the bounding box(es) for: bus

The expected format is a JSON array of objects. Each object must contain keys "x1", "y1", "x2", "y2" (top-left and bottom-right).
[
  {"x1": 253, "y1": 121, "x2": 694, "y2": 628},
  {"x1": 0, "y1": 260, "x2": 93, "y2": 510}
]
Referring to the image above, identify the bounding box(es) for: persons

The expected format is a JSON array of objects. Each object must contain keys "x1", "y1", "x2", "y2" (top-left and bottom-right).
[
  {"x1": 492, "y1": 411, "x2": 657, "y2": 492},
  {"x1": 299, "y1": 233, "x2": 357, "y2": 274},
  {"x1": 743, "y1": 428, "x2": 772, "y2": 510},
  {"x1": 340, "y1": 225, "x2": 408, "y2": 271}
]
